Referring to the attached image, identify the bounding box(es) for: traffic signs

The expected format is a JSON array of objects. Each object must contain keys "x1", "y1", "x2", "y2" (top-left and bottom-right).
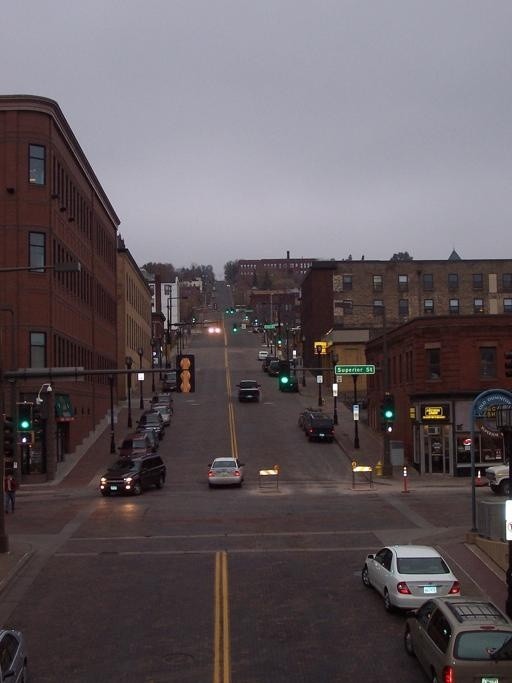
[{"x1": 335, "y1": 365, "x2": 376, "y2": 375}]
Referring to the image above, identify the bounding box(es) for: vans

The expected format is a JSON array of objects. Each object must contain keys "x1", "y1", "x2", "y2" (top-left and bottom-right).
[{"x1": 281, "y1": 376, "x2": 299, "y2": 392}]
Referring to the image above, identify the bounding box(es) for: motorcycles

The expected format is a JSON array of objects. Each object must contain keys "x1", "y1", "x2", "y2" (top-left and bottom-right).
[{"x1": 278, "y1": 372, "x2": 291, "y2": 392}]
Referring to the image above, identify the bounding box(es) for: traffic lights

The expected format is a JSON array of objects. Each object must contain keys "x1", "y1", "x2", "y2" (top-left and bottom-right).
[
  {"x1": 16, "y1": 432, "x2": 34, "y2": 445},
  {"x1": 2, "y1": 418, "x2": 15, "y2": 456},
  {"x1": 177, "y1": 354, "x2": 196, "y2": 393},
  {"x1": 382, "y1": 395, "x2": 395, "y2": 421},
  {"x1": 193, "y1": 317, "x2": 196, "y2": 324},
  {"x1": 17, "y1": 402, "x2": 32, "y2": 430},
  {"x1": 384, "y1": 423, "x2": 393, "y2": 433}
]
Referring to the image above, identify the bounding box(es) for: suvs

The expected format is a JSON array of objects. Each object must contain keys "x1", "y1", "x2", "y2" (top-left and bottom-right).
[
  {"x1": 486, "y1": 458, "x2": 511, "y2": 495},
  {"x1": 236, "y1": 378, "x2": 261, "y2": 403},
  {"x1": 405, "y1": 594, "x2": 512, "y2": 683},
  {"x1": 118, "y1": 392, "x2": 176, "y2": 459},
  {"x1": 163, "y1": 372, "x2": 177, "y2": 390},
  {"x1": 297, "y1": 406, "x2": 335, "y2": 443},
  {"x1": 99, "y1": 453, "x2": 166, "y2": 496}
]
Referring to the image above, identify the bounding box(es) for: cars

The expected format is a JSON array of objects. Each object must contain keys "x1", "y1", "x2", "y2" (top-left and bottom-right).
[
  {"x1": 362, "y1": 545, "x2": 462, "y2": 612},
  {"x1": 207, "y1": 457, "x2": 246, "y2": 488},
  {"x1": 251, "y1": 318, "x2": 262, "y2": 333},
  {"x1": 0, "y1": 627, "x2": 28, "y2": 682},
  {"x1": 257, "y1": 350, "x2": 282, "y2": 375}
]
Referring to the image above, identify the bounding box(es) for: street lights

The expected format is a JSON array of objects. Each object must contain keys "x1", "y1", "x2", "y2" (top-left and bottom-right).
[
  {"x1": 150, "y1": 337, "x2": 157, "y2": 392},
  {"x1": 301, "y1": 334, "x2": 307, "y2": 386},
  {"x1": 108, "y1": 374, "x2": 117, "y2": 454},
  {"x1": 262, "y1": 321, "x2": 289, "y2": 363},
  {"x1": 125, "y1": 356, "x2": 133, "y2": 427},
  {"x1": 158, "y1": 319, "x2": 190, "y2": 379},
  {"x1": 316, "y1": 344, "x2": 324, "y2": 406},
  {"x1": 166, "y1": 296, "x2": 188, "y2": 334},
  {"x1": 135, "y1": 347, "x2": 144, "y2": 410},
  {"x1": 293, "y1": 329, "x2": 297, "y2": 375}
]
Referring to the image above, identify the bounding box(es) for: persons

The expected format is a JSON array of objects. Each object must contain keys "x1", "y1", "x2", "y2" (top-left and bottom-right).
[{"x1": 4, "y1": 473, "x2": 17, "y2": 514}]
